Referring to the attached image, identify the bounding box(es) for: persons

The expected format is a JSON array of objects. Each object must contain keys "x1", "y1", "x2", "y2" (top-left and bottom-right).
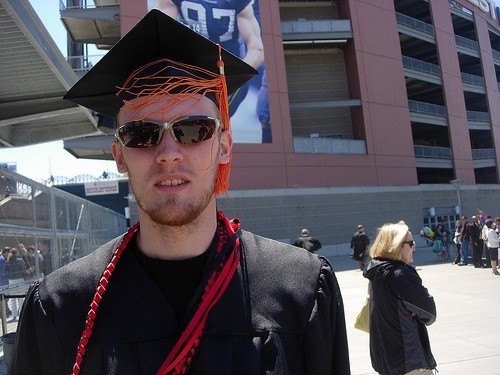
[
  {"x1": 8, "y1": 8, "x2": 352, "y2": 375},
  {"x1": 0, "y1": 240, "x2": 82, "y2": 323},
  {"x1": 362, "y1": 220, "x2": 439, "y2": 375},
  {"x1": 350, "y1": 224, "x2": 370, "y2": 271},
  {"x1": 421, "y1": 210, "x2": 500, "y2": 276},
  {"x1": 291, "y1": 229, "x2": 322, "y2": 253}
]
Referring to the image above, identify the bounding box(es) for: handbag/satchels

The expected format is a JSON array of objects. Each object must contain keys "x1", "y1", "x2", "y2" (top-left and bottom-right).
[
  {"x1": 351, "y1": 248, "x2": 364, "y2": 261},
  {"x1": 354, "y1": 279, "x2": 371, "y2": 333}
]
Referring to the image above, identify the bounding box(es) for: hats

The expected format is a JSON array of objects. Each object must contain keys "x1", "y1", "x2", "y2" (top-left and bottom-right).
[{"x1": 63, "y1": 9, "x2": 259, "y2": 116}]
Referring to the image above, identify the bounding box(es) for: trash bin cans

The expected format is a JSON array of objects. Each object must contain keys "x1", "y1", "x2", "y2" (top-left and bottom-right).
[{"x1": 1, "y1": 332, "x2": 19, "y2": 375}]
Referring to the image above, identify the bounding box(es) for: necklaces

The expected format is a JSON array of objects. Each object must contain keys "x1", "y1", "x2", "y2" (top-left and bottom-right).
[{"x1": 70, "y1": 212, "x2": 242, "y2": 375}]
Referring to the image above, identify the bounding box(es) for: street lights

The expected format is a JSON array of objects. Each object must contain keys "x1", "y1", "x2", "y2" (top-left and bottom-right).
[{"x1": 450, "y1": 179, "x2": 464, "y2": 220}]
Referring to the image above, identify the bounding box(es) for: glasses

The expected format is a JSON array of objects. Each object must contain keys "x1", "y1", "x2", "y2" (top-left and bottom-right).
[
  {"x1": 402, "y1": 240, "x2": 414, "y2": 248},
  {"x1": 114, "y1": 115, "x2": 224, "y2": 149}
]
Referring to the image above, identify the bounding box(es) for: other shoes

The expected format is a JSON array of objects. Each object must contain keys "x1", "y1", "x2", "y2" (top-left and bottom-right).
[{"x1": 452, "y1": 257, "x2": 500, "y2": 276}]
